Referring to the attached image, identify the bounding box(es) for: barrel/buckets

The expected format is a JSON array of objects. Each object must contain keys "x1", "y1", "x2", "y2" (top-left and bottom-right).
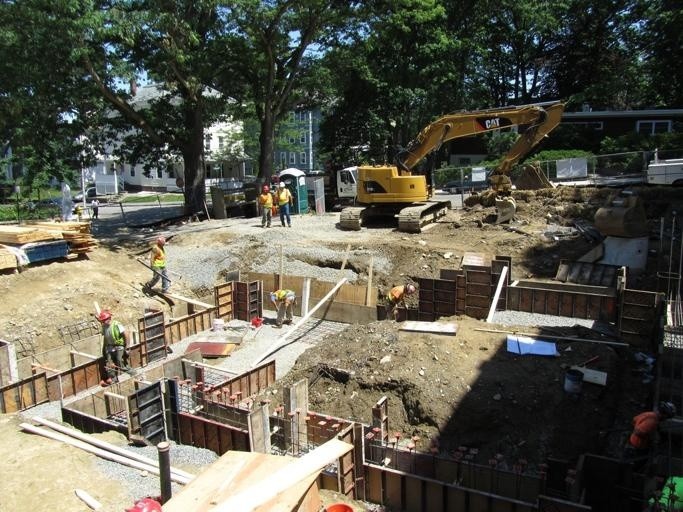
[
  {"x1": 213, "y1": 319, "x2": 224, "y2": 330},
  {"x1": 564, "y1": 367, "x2": 585, "y2": 393}
]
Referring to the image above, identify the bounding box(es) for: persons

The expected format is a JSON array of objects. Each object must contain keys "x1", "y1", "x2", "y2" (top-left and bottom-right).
[
  {"x1": 386, "y1": 284, "x2": 414, "y2": 320},
  {"x1": 259, "y1": 182, "x2": 293, "y2": 228},
  {"x1": 269, "y1": 290, "x2": 299, "y2": 328},
  {"x1": 97, "y1": 310, "x2": 131, "y2": 388},
  {"x1": 146, "y1": 238, "x2": 172, "y2": 295},
  {"x1": 90, "y1": 196, "x2": 100, "y2": 220},
  {"x1": 628, "y1": 402, "x2": 676, "y2": 474}
]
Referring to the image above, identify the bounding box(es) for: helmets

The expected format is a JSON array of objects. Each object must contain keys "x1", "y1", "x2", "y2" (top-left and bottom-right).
[
  {"x1": 158, "y1": 238, "x2": 164, "y2": 245},
  {"x1": 123, "y1": 497, "x2": 161, "y2": 512},
  {"x1": 660, "y1": 398, "x2": 676, "y2": 416},
  {"x1": 99, "y1": 310, "x2": 111, "y2": 321},
  {"x1": 407, "y1": 283, "x2": 415, "y2": 294},
  {"x1": 279, "y1": 181, "x2": 285, "y2": 187},
  {"x1": 262, "y1": 185, "x2": 268, "y2": 191}
]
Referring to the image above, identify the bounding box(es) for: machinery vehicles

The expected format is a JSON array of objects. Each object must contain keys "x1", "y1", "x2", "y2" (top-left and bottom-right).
[{"x1": 329, "y1": 98, "x2": 574, "y2": 235}]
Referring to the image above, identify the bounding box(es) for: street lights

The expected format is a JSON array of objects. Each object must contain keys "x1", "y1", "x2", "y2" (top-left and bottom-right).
[{"x1": 15, "y1": 184, "x2": 21, "y2": 221}]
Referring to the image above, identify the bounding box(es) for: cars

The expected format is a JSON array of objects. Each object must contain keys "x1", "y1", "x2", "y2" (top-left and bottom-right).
[
  {"x1": 73, "y1": 189, "x2": 88, "y2": 202},
  {"x1": 27, "y1": 196, "x2": 76, "y2": 218}
]
